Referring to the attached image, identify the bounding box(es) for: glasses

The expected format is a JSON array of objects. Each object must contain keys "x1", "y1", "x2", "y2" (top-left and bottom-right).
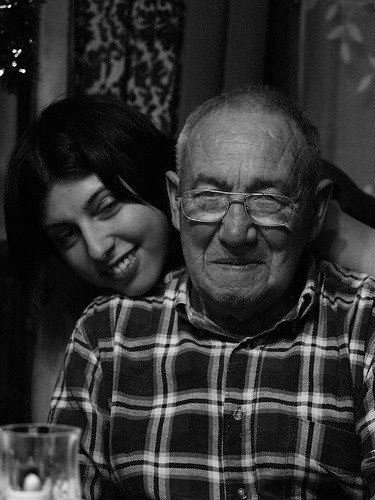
[{"x1": 176, "y1": 181, "x2": 316, "y2": 227}]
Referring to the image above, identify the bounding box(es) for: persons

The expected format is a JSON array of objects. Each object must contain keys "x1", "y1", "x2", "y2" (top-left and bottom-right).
[
  {"x1": 8, "y1": 90, "x2": 375, "y2": 432},
  {"x1": 39, "y1": 84, "x2": 375, "y2": 499}
]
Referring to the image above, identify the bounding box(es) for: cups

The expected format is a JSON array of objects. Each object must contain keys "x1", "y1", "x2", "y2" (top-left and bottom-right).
[{"x1": 0, "y1": 423, "x2": 82, "y2": 500}]
